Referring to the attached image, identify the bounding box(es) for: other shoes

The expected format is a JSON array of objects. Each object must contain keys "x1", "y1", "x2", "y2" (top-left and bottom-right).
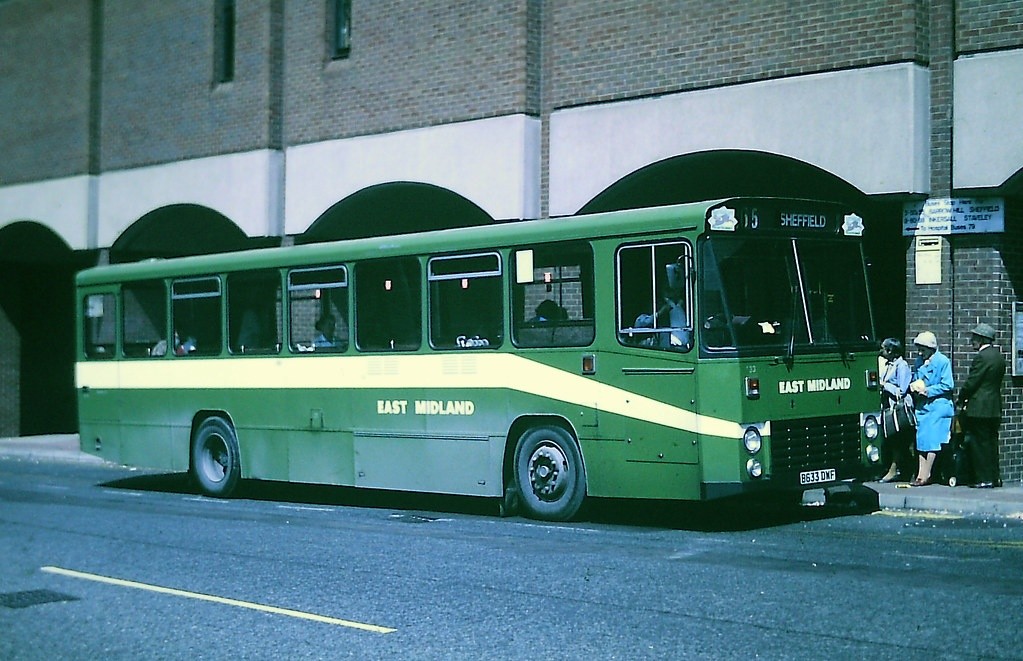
[
  {"x1": 909, "y1": 478, "x2": 932, "y2": 486},
  {"x1": 878, "y1": 474, "x2": 899, "y2": 482}
]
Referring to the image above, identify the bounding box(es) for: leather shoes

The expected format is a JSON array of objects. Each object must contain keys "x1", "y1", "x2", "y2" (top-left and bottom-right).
[
  {"x1": 969, "y1": 481, "x2": 994, "y2": 488},
  {"x1": 993, "y1": 478, "x2": 1002, "y2": 487}
]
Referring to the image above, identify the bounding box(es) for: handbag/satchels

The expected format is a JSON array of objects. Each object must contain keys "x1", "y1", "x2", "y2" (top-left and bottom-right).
[{"x1": 882, "y1": 388, "x2": 919, "y2": 437}]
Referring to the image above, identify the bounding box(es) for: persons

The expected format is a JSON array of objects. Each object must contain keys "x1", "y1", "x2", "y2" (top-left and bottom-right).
[
  {"x1": 909, "y1": 330, "x2": 955, "y2": 486},
  {"x1": 310, "y1": 313, "x2": 344, "y2": 349},
  {"x1": 526, "y1": 299, "x2": 569, "y2": 325},
  {"x1": 957, "y1": 323, "x2": 1006, "y2": 490},
  {"x1": 872, "y1": 337, "x2": 912, "y2": 481},
  {"x1": 152, "y1": 320, "x2": 196, "y2": 359},
  {"x1": 633, "y1": 283, "x2": 687, "y2": 348}
]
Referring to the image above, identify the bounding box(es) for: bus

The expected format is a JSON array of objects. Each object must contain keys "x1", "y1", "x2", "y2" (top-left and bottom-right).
[{"x1": 74, "y1": 196, "x2": 886, "y2": 524}]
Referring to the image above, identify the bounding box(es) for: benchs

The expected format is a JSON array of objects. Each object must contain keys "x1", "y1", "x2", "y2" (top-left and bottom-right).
[{"x1": 517, "y1": 317, "x2": 595, "y2": 347}]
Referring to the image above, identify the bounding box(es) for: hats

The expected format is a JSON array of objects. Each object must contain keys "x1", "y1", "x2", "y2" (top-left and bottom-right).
[
  {"x1": 971, "y1": 322, "x2": 996, "y2": 341},
  {"x1": 914, "y1": 331, "x2": 938, "y2": 350}
]
